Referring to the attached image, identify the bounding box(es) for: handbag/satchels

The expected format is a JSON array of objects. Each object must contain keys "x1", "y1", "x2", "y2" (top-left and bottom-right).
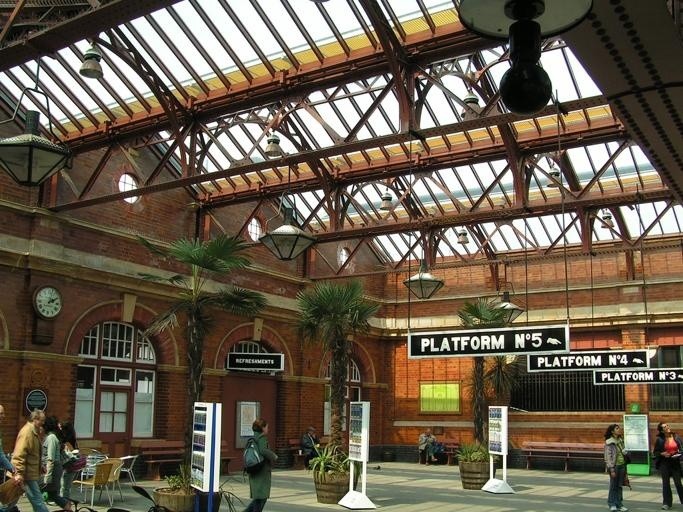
[
  {"x1": 67, "y1": 454, "x2": 85, "y2": 472},
  {"x1": 60, "y1": 444, "x2": 77, "y2": 465},
  {"x1": 652, "y1": 456, "x2": 661, "y2": 470},
  {"x1": 623, "y1": 471, "x2": 632, "y2": 490},
  {"x1": 624, "y1": 451, "x2": 631, "y2": 463}
]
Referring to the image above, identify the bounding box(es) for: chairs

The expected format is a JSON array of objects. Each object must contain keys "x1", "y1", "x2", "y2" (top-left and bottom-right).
[{"x1": 71, "y1": 446, "x2": 136, "y2": 509}]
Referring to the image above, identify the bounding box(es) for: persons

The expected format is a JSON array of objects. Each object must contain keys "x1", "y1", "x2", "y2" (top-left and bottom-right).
[
  {"x1": 652, "y1": 423, "x2": 682, "y2": 509},
  {"x1": 302, "y1": 425, "x2": 321, "y2": 468},
  {"x1": 241, "y1": 418, "x2": 278, "y2": 511},
  {"x1": 0, "y1": 405, "x2": 18, "y2": 512},
  {"x1": 58, "y1": 421, "x2": 78, "y2": 498},
  {"x1": 39, "y1": 418, "x2": 71, "y2": 510},
  {"x1": 603, "y1": 424, "x2": 630, "y2": 511},
  {"x1": 4, "y1": 409, "x2": 50, "y2": 511},
  {"x1": 418, "y1": 427, "x2": 437, "y2": 465}
]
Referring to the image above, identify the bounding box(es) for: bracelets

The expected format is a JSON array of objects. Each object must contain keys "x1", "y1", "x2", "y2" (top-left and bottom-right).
[{"x1": 662, "y1": 452, "x2": 665, "y2": 456}]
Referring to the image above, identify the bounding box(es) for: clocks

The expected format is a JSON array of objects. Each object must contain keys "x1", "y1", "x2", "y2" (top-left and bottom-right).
[{"x1": 30, "y1": 283, "x2": 64, "y2": 346}]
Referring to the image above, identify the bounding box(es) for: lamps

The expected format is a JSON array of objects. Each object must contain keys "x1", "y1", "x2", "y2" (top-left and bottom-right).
[
  {"x1": 79, "y1": 29, "x2": 105, "y2": 83},
  {"x1": 545, "y1": 151, "x2": 562, "y2": 189},
  {"x1": 378, "y1": 183, "x2": 394, "y2": 210},
  {"x1": 288, "y1": 276, "x2": 382, "y2": 505},
  {"x1": 401, "y1": 229, "x2": 446, "y2": 301},
  {"x1": 599, "y1": 206, "x2": 614, "y2": 230},
  {"x1": 256, "y1": 164, "x2": 316, "y2": 263},
  {"x1": 134, "y1": 229, "x2": 272, "y2": 512},
  {"x1": 458, "y1": 81, "x2": 482, "y2": 121},
  {"x1": 0, "y1": 54, "x2": 74, "y2": 191},
  {"x1": 456, "y1": 224, "x2": 470, "y2": 245},
  {"x1": 263, "y1": 125, "x2": 283, "y2": 158},
  {"x1": 486, "y1": 260, "x2": 527, "y2": 326},
  {"x1": 449, "y1": 294, "x2": 508, "y2": 492},
  {"x1": 454, "y1": 1, "x2": 595, "y2": 118}
]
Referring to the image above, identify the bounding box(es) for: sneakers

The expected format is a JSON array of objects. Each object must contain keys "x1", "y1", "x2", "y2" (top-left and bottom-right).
[
  {"x1": 617, "y1": 506, "x2": 628, "y2": 512},
  {"x1": 608, "y1": 505, "x2": 617, "y2": 511},
  {"x1": 661, "y1": 504, "x2": 672, "y2": 510}
]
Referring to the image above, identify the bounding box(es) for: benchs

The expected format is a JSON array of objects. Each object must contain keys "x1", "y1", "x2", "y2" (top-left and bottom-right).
[
  {"x1": 288, "y1": 438, "x2": 347, "y2": 471},
  {"x1": 519, "y1": 441, "x2": 609, "y2": 474},
  {"x1": 418, "y1": 441, "x2": 461, "y2": 467},
  {"x1": 136, "y1": 438, "x2": 240, "y2": 482}
]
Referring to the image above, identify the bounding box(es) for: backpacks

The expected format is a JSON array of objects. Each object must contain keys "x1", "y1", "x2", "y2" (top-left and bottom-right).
[{"x1": 243, "y1": 434, "x2": 267, "y2": 475}]
